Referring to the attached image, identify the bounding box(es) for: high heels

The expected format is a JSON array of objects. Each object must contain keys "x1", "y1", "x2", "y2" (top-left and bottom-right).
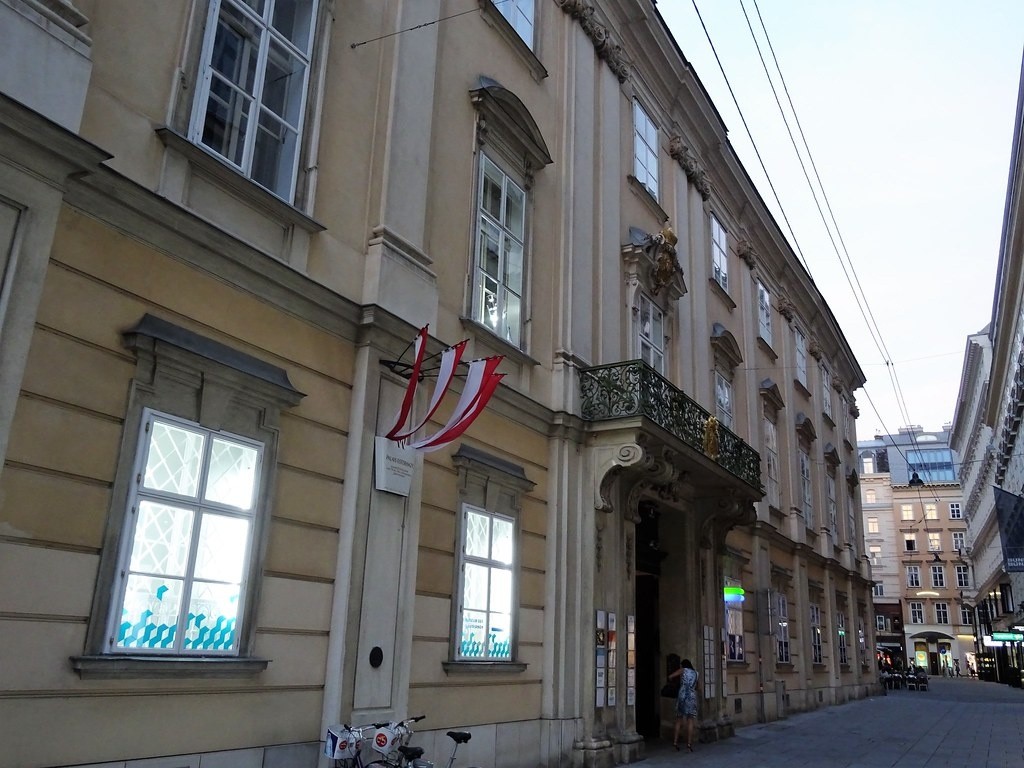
[
  {"x1": 672, "y1": 744, "x2": 679, "y2": 752},
  {"x1": 687, "y1": 743, "x2": 694, "y2": 752}
]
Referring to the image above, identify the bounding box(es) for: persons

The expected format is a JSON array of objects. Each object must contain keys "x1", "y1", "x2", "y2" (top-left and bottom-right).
[
  {"x1": 668, "y1": 658, "x2": 703, "y2": 753},
  {"x1": 953, "y1": 658, "x2": 963, "y2": 678},
  {"x1": 877, "y1": 654, "x2": 928, "y2": 691},
  {"x1": 966, "y1": 660, "x2": 976, "y2": 677},
  {"x1": 910, "y1": 472, "x2": 924, "y2": 486},
  {"x1": 942, "y1": 655, "x2": 953, "y2": 679}
]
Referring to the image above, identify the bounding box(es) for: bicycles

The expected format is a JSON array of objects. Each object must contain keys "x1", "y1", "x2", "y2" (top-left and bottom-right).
[
  {"x1": 392, "y1": 714, "x2": 487, "y2": 768},
  {"x1": 324, "y1": 721, "x2": 426, "y2": 768}
]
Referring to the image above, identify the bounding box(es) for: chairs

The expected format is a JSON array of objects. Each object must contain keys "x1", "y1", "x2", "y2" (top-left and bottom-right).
[{"x1": 884, "y1": 679, "x2": 928, "y2": 692}]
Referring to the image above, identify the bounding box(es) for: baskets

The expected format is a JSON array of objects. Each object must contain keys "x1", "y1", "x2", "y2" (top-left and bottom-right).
[
  {"x1": 372, "y1": 721, "x2": 408, "y2": 756},
  {"x1": 323, "y1": 725, "x2": 363, "y2": 759}
]
